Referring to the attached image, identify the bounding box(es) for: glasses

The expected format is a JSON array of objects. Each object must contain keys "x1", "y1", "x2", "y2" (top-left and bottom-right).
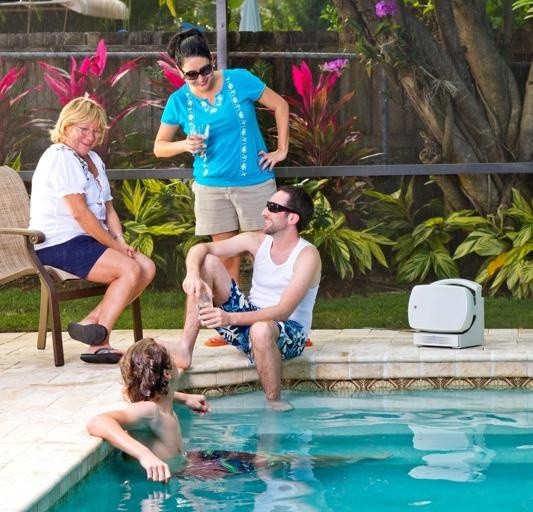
[
  {"x1": 266, "y1": 200, "x2": 299, "y2": 215},
  {"x1": 72, "y1": 124, "x2": 102, "y2": 137},
  {"x1": 176, "y1": 59, "x2": 212, "y2": 81}
]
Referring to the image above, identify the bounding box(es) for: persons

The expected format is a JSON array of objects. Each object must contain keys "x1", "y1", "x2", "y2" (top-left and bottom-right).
[
  {"x1": 28, "y1": 97, "x2": 155, "y2": 363},
  {"x1": 140, "y1": 489, "x2": 314, "y2": 511},
  {"x1": 152, "y1": 24, "x2": 314, "y2": 348},
  {"x1": 154, "y1": 184, "x2": 323, "y2": 412},
  {"x1": 86, "y1": 338, "x2": 392, "y2": 484}
]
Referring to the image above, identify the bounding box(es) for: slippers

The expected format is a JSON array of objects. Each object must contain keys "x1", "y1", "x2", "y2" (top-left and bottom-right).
[
  {"x1": 204, "y1": 335, "x2": 228, "y2": 347},
  {"x1": 78, "y1": 348, "x2": 123, "y2": 364},
  {"x1": 67, "y1": 323, "x2": 107, "y2": 346}
]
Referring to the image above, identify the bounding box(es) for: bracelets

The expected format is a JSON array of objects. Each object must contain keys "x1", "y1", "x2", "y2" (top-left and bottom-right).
[{"x1": 113, "y1": 234, "x2": 124, "y2": 240}]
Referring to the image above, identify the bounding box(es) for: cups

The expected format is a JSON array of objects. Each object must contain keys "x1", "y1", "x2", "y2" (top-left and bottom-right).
[
  {"x1": 189, "y1": 124, "x2": 209, "y2": 158},
  {"x1": 195, "y1": 291, "x2": 213, "y2": 316}
]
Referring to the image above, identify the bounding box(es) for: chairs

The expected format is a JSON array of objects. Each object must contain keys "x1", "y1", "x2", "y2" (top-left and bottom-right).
[{"x1": 0, "y1": 165, "x2": 144, "y2": 368}]
